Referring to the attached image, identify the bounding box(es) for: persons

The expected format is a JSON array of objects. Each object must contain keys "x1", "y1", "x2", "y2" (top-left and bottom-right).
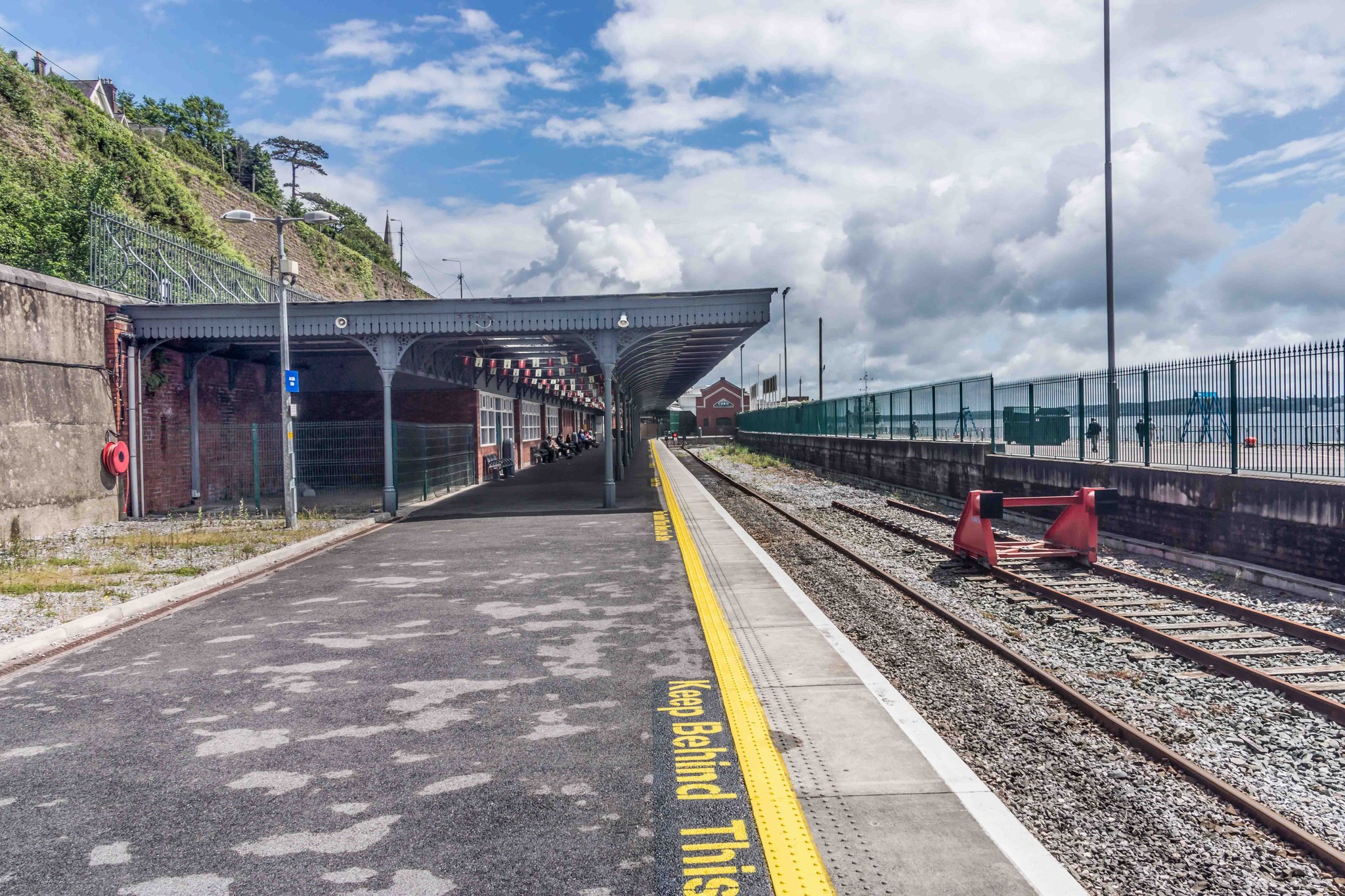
[
  {"x1": 578, "y1": 429, "x2": 600, "y2": 450},
  {"x1": 828, "y1": 423, "x2": 833, "y2": 434},
  {"x1": 547, "y1": 434, "x2": 573, "y2": 460},
  {"x1": 1149, "y1": 418, "x2": 1156, "y2": 446},
  {"x1": 556, "y1": 432, "x2": 579, "y2": 456},
  {"x1": 566, "y1": 434, "x2": 582, "y2": 453},
  {"x1": 540, "y1": 436, "x2": 557, "y2": 463},
  {"x1": 912, "y1": 420, "x2": 919, "y2": 438},
  {"x1": 1136, "y1": 418, "x2": 1144, "y2": 446},
  {"x1": 1088, "y1": 418, "x2": 1102, "y2": 452}
]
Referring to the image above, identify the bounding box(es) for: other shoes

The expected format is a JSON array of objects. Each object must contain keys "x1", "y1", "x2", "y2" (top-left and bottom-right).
[
  {"x1": 555, "y1": 458, "x2": 559, "y2": 461},
  {"x1": 558, "y1": 457, "x2": 563, "y2": 459},
  {"x1": 595, "y1": 444, "x2": 599, "y2": 448},
  {"x1": 548, "y1": 460, "x2": 556, "y2": 463},
  {"x1": 566, "y1": 456, "x2": 573, "y2": 459}
]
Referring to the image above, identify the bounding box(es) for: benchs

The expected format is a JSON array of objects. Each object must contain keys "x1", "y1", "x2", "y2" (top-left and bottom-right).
[
  {"x1": 530, "y1": 443, "x2": 586, "y2": 463},
  {"x1": 484, "y1": 454, "x2": 515, "y2": 481},
  {"x1": 1309, "y1": 441, "x2": 1345, "y2": 451}
]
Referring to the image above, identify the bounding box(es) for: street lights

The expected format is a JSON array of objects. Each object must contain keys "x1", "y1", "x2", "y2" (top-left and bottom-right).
[
  {"x1": 220, "y1": 209, "x2": 342, "y2": 530},
  {"x1": 390, "y1": 218, "x2": 403, "y2": 277},
  {"x1": 443, "y1": 259, "x2": 463, "y2": 299},
  {"x1": 740, "y1": 344, "x2": 745, "y2": 411},
  {"x1": 782, "y1": 287, "x2": 791, "y2": 407}
]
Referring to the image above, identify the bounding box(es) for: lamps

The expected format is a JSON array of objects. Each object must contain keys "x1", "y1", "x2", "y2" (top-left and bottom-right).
[
  {"x1": 617, "y1": 312, "x2": 629, "y2": 327},
  {"x1": 336, "y1": 318, "x2": 348, "y2": 328},
  {"x1": 456, "y1": 312, "x2": 494, "y2": 335}
]
